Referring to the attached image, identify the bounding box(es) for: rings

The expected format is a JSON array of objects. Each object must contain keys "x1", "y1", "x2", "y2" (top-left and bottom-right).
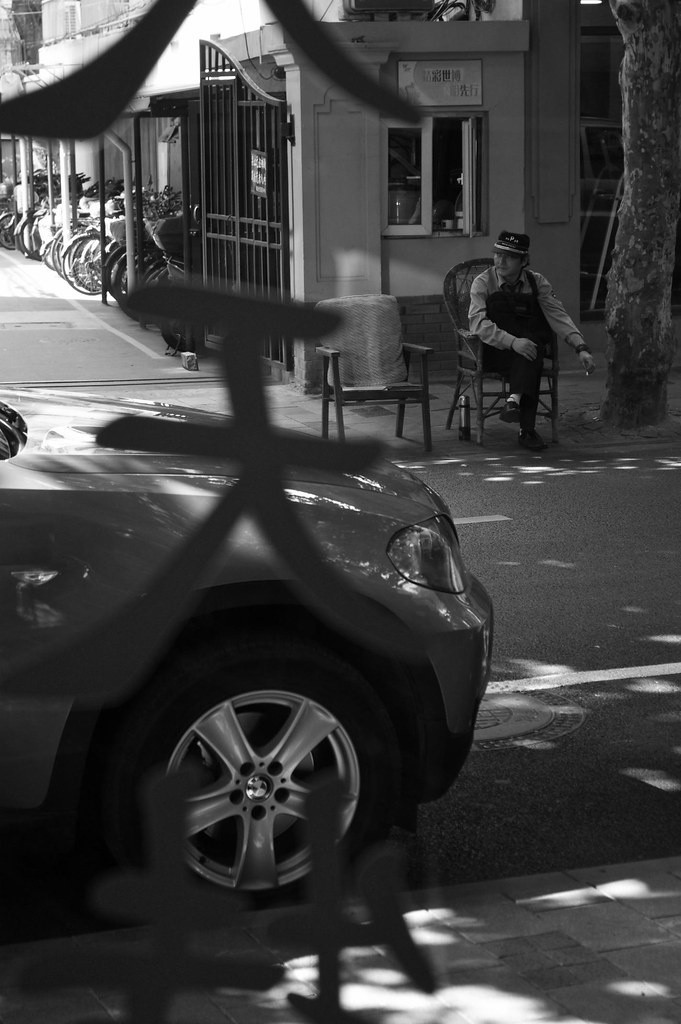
[{"x1": 593, "y1": 363, "x2": 596, "y2": 369}]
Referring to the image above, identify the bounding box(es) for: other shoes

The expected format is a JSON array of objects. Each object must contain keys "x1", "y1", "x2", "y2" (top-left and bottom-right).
[
  {"x1": 500, "y1": 402, "x2": 521, "y2": 424},
  {"x1": 518, "y1": 429, "x2": 547, "y2": 449}
]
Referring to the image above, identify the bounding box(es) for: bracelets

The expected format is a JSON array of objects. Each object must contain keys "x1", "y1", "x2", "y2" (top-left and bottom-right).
[{"x1": 575, "y1": 343, "x2": 592, "y2": 355}]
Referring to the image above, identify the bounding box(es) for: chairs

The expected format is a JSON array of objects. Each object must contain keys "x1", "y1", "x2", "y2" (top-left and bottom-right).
[
  {"x1": 443, "y1": 259, "x2": 563, "y2": 450},
  {"x1": 314, "y1": 294, "x2": 436, "y2": 453}
]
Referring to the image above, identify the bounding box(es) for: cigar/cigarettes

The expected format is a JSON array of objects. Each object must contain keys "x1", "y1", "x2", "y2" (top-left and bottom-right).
[{"x1": 586, "y1": 371, "x2": 589, "y2": 376}]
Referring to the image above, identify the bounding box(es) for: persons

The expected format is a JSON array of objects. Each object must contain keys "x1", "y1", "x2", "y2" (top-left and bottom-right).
[{"x1": 467, "y1": 229, "x2": 596, "y2": 451}]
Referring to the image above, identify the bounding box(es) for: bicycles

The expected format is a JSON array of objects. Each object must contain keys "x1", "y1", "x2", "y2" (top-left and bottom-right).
[{"x1": 0, "y1": 170, "x2": 197, "y2": 356}]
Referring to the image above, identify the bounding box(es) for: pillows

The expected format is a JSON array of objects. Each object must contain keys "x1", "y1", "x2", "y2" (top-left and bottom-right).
[{"x1": 316, "y1": 295, "x2": 408, "y2": 387}]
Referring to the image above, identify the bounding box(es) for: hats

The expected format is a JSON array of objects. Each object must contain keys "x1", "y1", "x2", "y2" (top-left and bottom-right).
[{"x1": 491, "y1": 230, "x2": 531, "y2": 258}]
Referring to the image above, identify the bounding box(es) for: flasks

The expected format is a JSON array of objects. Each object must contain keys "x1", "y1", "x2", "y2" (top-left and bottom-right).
[{"x1": 458, "y1": 395, "x2": 471, "y2": 441}]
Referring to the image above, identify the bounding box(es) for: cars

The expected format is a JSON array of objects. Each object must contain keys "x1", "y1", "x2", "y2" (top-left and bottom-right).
[
  {"x1": 0, "y1": 387, "x2": 493, "y2": 909},
  {"x1": 389, "y1": 148, "x2": 421, "y2": 220},
  {"x1": 580, "y1": 115, "x2": 624, "y2": 250}
]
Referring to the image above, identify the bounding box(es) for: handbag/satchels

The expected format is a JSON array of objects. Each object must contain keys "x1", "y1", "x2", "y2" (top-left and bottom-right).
[{"x1": 487, "y1": 271, "x2": 554, "y2": 347}]
